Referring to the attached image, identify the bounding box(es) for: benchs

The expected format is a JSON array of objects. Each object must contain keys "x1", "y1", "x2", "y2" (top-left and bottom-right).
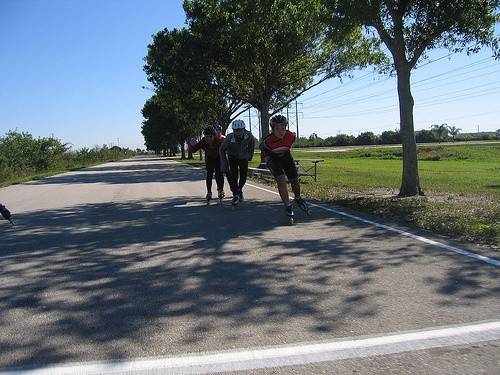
[{"x1": 248, "y1": 158, "x2": 324, "y2": 185}]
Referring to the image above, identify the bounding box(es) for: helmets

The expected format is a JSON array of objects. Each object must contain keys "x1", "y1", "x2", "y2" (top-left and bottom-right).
[
  {"x1": 232, "y1": 119, "x2": 245, "y2": 130},
  {"x1": 204, "y1": 127, "x2": 217, "y2": 136},
  {"x1": 270, "y1": 115, "x2": 288, "y2": 127}
]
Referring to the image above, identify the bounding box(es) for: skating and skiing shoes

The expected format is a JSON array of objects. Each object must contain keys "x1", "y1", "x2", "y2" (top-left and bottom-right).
[
  {"x1": 295, "y1": 198, "x2": 310, "y2": 216},
  {"x1": 232, "y1": 196, "x2": 239, "y2": 209},
  {"x1": 218, "y1": 190, "x2": 225, "y2": 204},
  {"x1": 238, "y1": 189, "x2": 244, "y2": 203},
  {"x1": 285, "y1": 203, "x2": 295, "y2": 225},
  {"x1": 207, "y1": 193, "x2": 212, "y2": 206},
  {"x1": 0, "y1": 204, "x2": 15, "y2": 225}
]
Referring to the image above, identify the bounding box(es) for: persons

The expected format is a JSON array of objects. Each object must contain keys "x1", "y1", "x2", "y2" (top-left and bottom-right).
[
  {"x1": 225, "y1": 119, "x2": 256, "y2": 208},
  {"x1": 0, "y1": 204, "x2": 16, "y2": 225},
  {"x1": 259, "y1": 115, "x2": 311, "y2": 224},
  {"x1": 189, "y1": 124, "x2": 231, "y2": 206}
]
